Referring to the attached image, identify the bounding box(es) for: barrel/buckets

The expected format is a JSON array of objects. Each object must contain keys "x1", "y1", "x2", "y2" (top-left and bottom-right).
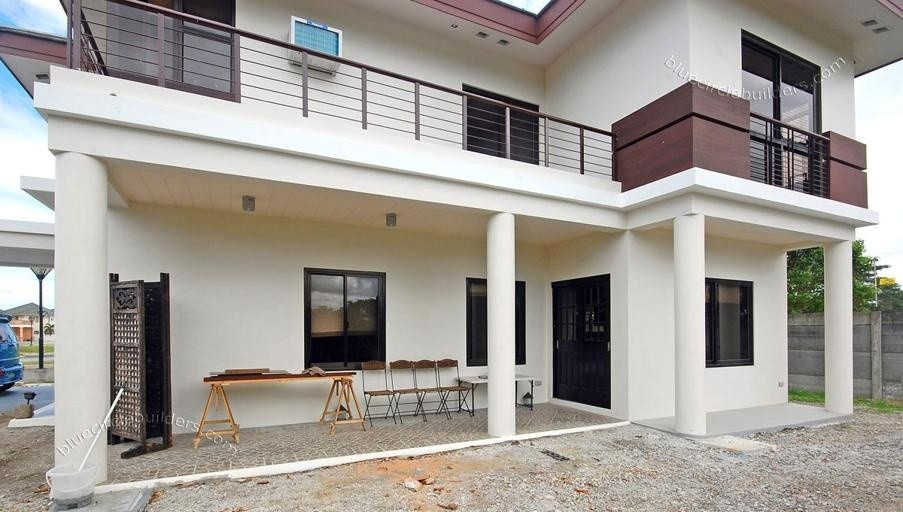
[{"x1": 45, "y1": 462, "x2": 97, "y2": 509}]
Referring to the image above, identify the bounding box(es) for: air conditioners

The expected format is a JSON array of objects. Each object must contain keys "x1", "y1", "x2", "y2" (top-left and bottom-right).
[{"x1": 285, "y1": 15, "x2": 343, "y2": 75}]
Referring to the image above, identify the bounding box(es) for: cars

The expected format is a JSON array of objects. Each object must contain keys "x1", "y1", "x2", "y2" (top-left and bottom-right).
[{"x1": 0, "y1": 312, "x2": 23, "y2": 394}]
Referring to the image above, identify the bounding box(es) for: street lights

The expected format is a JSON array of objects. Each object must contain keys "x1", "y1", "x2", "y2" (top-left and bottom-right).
[{"x1": 29, "y1": 265, "x2": 54, "y2": 369}]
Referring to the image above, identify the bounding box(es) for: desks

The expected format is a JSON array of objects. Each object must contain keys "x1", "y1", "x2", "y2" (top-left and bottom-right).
[
  {"x1": 456, "y1": 374, "x2": 536, "y2": 416},
  {"x1": 192, "y1": 370, "x2": 367, "y2": 448}
]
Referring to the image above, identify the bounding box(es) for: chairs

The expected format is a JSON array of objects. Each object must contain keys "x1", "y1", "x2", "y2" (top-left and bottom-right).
[{"x1": 361, "y1": 359, "x2": 470, "y2": 427}]
